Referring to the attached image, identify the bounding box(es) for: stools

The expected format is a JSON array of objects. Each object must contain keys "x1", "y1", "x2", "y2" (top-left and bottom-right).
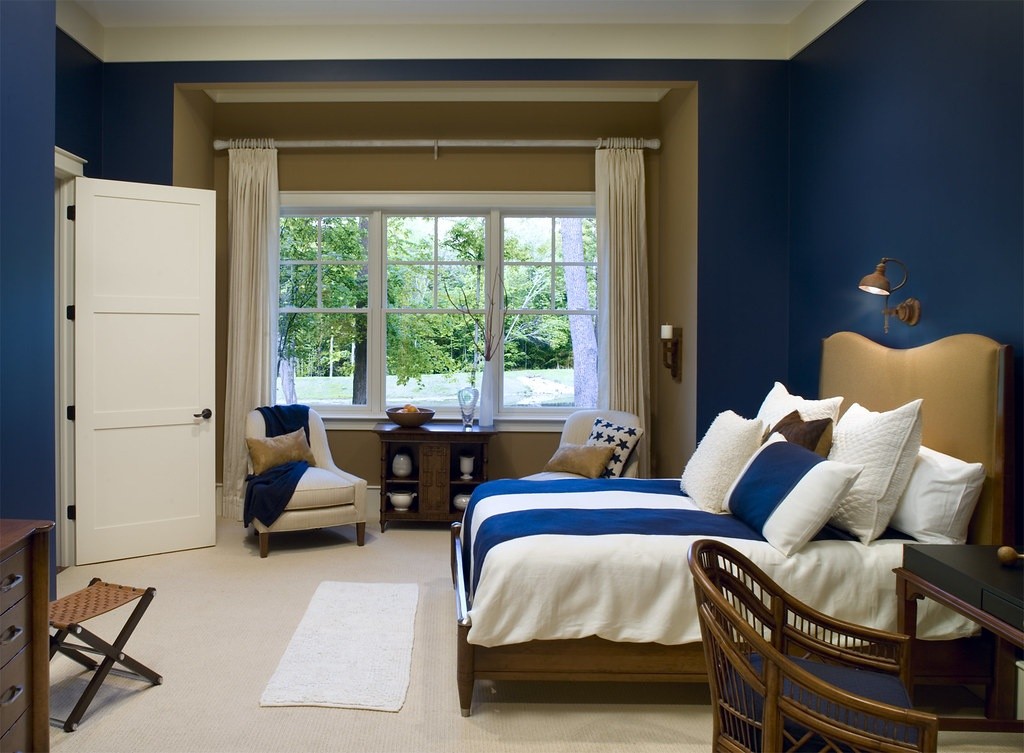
[{"x1": 50, "y1": 577, "x2": 163, "y2": 732}]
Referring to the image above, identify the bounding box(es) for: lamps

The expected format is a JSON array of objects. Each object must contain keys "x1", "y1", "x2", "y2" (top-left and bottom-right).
[{"x1": 859, "y1": 258, "x2": 920, "y2": 334}]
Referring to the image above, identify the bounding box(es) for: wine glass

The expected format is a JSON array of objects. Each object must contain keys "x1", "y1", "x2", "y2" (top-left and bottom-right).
[{"x1": 460, "y1": 457, "x2": 474, "y2": 479}]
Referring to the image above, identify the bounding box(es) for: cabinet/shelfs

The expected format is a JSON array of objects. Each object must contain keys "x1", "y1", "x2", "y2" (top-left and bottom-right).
[
  {"x1": 372, "y1": 422, "x2": 498, "y2": 534},
  {"x1": 0, "y1": 518, "x2": 56, "y2": 753}
]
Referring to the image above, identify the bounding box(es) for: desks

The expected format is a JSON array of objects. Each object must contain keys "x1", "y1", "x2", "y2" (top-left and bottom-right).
[{"x1": 890, "y1": 543, "x2": 1024, "y2": 731}]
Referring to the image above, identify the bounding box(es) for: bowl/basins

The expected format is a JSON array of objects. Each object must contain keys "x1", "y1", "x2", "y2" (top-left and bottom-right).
[
  {"x1": 387, "y1": 491, "x2": 417, "y2": 510},
  {"x1": 385, "y1": 407, "x2": 435, "y2": 427}
]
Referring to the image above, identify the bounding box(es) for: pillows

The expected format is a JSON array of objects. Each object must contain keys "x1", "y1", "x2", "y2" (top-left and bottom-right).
[
  {"x1": 889, "y1": 445, "x2": 987, "y2": 545},
  {"x1": 760, "y1": 410, "x2": 834, "y2": 458},
  {"x1": 543, "y1": 443, "x2": 617, "y2": 479},
  {"x1": 244, "y1": 427, "x2": 317, "y2": 476},
  {"x1": 585, "y1": 417, "x2": 644, "y2": 479},
  {"x1": 720, "y1": 431, "x2": 866, "y2": 557},
  {"x1": 757, "y1": 381, "x2": 844, "y2": 435},
  {"x1": 827, "y1": 398, "x2": 925, "y2": 546},
  {"x1": 679, "y1": 410, "x2": 770, "y2": 513}
]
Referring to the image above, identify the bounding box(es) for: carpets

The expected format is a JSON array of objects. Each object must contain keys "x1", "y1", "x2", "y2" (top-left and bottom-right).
[{"x1": 259, "y1": 580, "x2": 419, "y2": 713}]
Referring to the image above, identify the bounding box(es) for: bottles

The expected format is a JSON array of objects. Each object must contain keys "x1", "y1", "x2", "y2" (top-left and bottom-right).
[{"x1": 458, "y1": 386, "x2": 480, "y2": 428}]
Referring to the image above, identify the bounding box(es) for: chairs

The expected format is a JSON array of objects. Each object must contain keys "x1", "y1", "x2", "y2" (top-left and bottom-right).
[
  {"x1": 244, "y1": 406, "x2": 368, "y2": 559},
  {"x1": 518, "y1": 409, "x2": 641, "y2": 479},
  {"x1": 687, "y1": 539, "x2": 940, "y2": 753}
]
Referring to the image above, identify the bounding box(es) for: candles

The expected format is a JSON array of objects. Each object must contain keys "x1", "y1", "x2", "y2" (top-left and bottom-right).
[{"x1": 661, "y1": 322, "x2": 673, "y2": 339}]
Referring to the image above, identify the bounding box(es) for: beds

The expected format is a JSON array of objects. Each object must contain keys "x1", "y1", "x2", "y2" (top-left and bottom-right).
[{"x1": 447, "y1": 330, "x2": 1019, "y2": 720}]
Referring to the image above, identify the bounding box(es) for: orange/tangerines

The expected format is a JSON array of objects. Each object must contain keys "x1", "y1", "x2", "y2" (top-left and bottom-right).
[{"x1": 404, "y1": 403, "x2": 419, "y2": 413}]
